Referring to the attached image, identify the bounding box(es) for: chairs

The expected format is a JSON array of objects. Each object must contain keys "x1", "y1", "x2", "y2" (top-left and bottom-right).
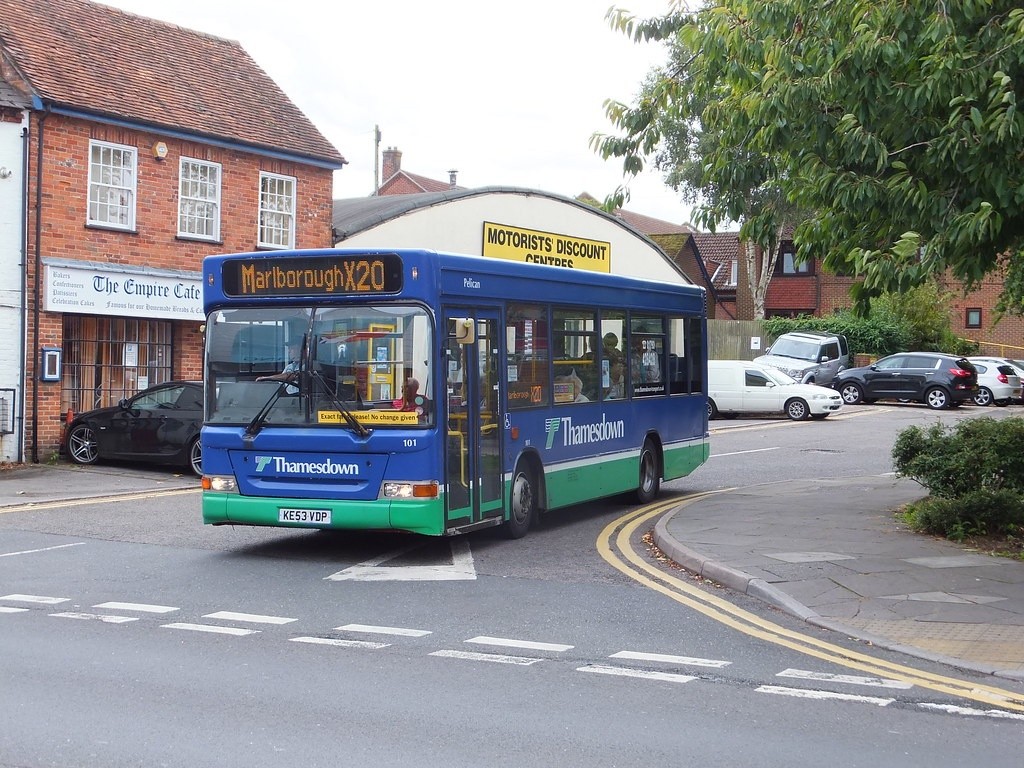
[
  {"x1": 659, "y1": 353, "x2": 678, "y2": 382},
  {"x1": 518, "y1": 354, "x2": 548, "y2": 386},
  {"x1": 320, "y1": 359, "x2": 336, "y2": 397},
  {"x1": 507, "y1": 353, "x2": 522, "y2": 384}
]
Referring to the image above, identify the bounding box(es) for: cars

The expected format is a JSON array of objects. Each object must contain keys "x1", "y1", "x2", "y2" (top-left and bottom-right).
[
  {"x1": 62, "y1": 378, "x2": 206, "y2": 477},
  {"x1": 953, "y1": 356, "x2": 1024, "y2": 407}
]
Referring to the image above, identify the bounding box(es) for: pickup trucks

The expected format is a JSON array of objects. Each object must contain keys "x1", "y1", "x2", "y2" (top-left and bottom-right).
[{"x1": 706, "y1": 360, "x2": 845, "y2": 421}]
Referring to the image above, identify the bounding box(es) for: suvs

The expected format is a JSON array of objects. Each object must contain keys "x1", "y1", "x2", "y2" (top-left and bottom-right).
[
  {"x1": 752, "y1": 330, "x2": 853, "y2": 389},
  {"x1": 835, "y1": 352, "x2": 978, "y2": 411}
]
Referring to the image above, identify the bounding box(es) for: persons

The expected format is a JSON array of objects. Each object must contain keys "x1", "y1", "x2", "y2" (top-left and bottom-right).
[
  {"x1": 578, "y1": 327, "x2": 665, "y2": 401},
  {"x1": 554, "y1": 374, "x2": 590, "y2": 402},
  {"x1": 456, "y1": 351, "x2": 487, "y2": 383},
  {"x1": 255, "y1": 336, "x2": 321, "y2": 397},
  {"x1": 391, "y1": 378, "x2": 428, "y2": 420}
]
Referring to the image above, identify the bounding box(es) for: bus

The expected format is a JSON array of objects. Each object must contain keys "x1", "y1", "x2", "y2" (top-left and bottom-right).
[{"x1": 199, "y1": 247, "x2": 710, "y2": 539}]
